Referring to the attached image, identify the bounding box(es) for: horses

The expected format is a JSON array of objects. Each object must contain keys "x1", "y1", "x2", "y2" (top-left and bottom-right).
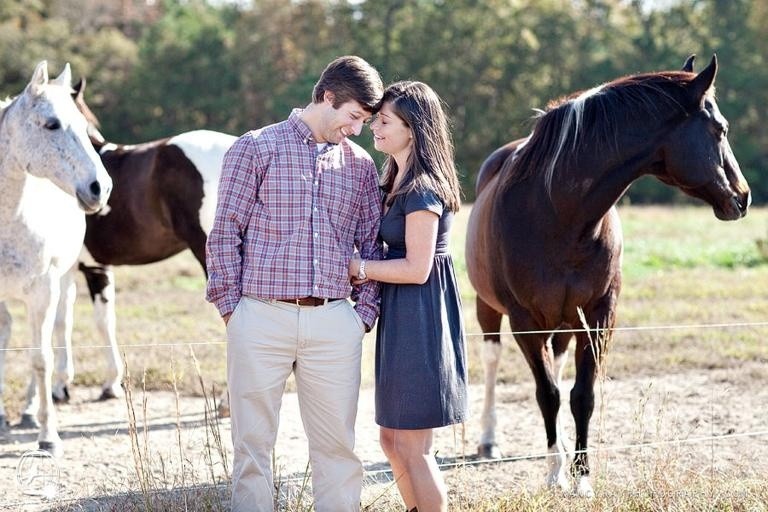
[
  {"x1": 14, "y1": 73, "x2": 238, "y2": 419},
  {"x1": 0, "y1": 59, "x2": 113, "y2": 458},
  {"x1": 465, "y1": 53, "x2": 752, "y2": 500}
]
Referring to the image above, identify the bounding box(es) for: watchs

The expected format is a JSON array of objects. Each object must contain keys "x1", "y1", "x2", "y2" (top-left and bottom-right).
[{"x1": 359, "y1": 257, "x2": 367, "y2": 279}]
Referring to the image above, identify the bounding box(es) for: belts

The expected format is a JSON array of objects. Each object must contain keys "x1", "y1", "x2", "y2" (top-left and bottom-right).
[{"x1": 277, "y1": 295, "x2": 347, "y2": 307}]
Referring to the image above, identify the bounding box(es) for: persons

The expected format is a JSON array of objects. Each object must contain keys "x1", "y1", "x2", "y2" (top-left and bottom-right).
[
  {"x1": 349, "y1": 77, "x2": 470, "y2": 511},
  {"x1": 196, "y1": 53, "x2": 386, "y2": 511}
]
